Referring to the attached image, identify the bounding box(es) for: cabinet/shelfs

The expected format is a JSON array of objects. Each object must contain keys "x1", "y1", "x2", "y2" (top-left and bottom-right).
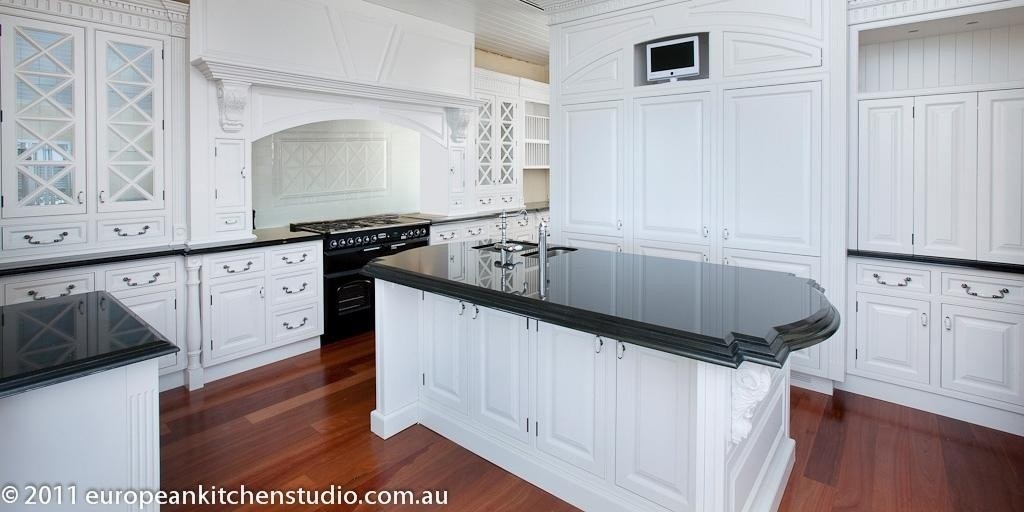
[
  {"x1": 470, "y1": 91, "x2": 521, "y2": 218},
  {"x1": 0, "y1": 5, "x2": 171, "y2": 265},
  {"x1": 854, "y1": 88, "x2": 913, "y2": 256},
  {"x1": 557, "y1": 72, "x2": 831, "y2": 395},
  {"x1": 843, "y1": 250, "x2": 1024, "y2": 438},
  {"x1": 199, "y1": 239, "x2": 324, "y2": 386},
  {"x1": 419, "y1": 291, "x2": 796, "y2": 512},
  {"x1": 211, "y1": 136, "x2": 248, "y2": 241},
  {"x1": 912, "y1": 81, "x2": 1023, "y2": 266},
  {"x1": 0, "y1": 253, "x2": 185, "y2": 393},
  {"x1": 428, "y1": 209, "x2": 549, "y2": 246},
  {"x1": 419, "y1": 147, "x2": 468, "y2": 217}
]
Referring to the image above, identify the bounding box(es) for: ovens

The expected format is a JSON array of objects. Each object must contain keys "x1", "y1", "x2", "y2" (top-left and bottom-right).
[{"x1": 323, "y1": 224, "x2": 430, "y2": 344}]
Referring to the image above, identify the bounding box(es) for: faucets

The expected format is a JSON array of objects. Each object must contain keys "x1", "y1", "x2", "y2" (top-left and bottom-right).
[
  {"x1": 495, "y1": 249, "x2": 528, "y2": 296},
  {"x1": 539, "y1": 257, "x2": 550, "y2": 301},
  {"x1": 537, "y1": 215, "x2": 551, "y2": 258},
  {"x1": 499, "y1": 207, "x2": 530, "y2": 246}
]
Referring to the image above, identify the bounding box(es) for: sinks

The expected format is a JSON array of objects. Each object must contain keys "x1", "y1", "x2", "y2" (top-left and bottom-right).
[
  {"x1": 519, "y1": 247, "x2": 578, "y2": 258},
  {"x1": 471, "y1": 238, "x2": 540, "y2": 252}
]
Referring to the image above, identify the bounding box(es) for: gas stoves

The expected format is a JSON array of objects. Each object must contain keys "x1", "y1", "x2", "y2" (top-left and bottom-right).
[{"x1": 290, "y1": 214, "x2": 432, "y2": 234}]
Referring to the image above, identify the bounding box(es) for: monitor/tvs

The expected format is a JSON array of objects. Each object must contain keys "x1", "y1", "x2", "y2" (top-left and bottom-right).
[{"x1": 647, "y1": 36, "x2": 700, "y2": 82}]
